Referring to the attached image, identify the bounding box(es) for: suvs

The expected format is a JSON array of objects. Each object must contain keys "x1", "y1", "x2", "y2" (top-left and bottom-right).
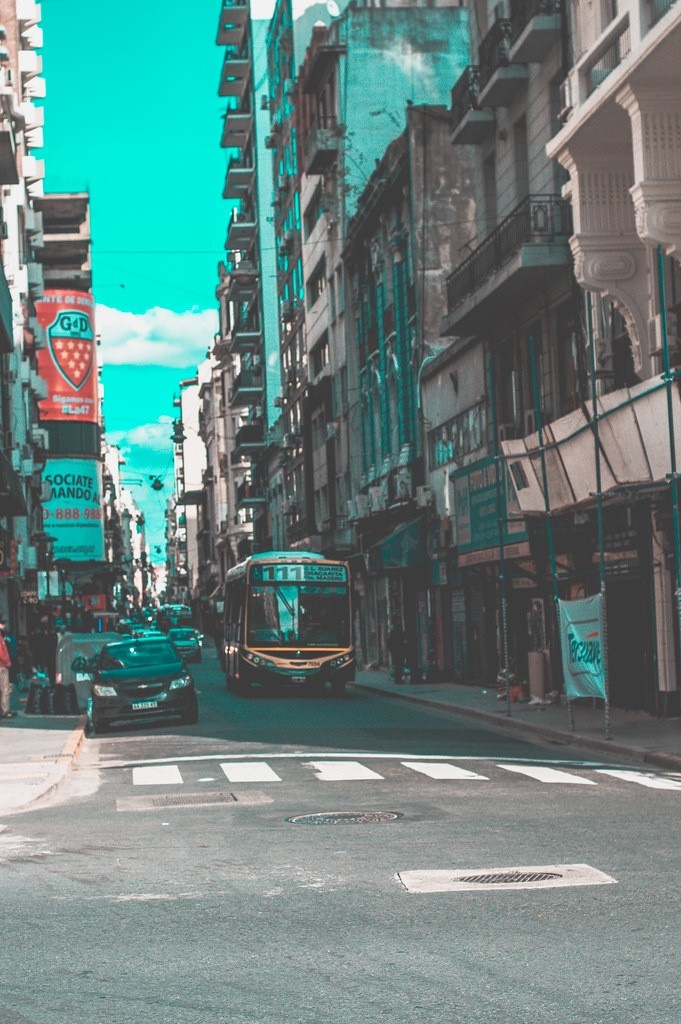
[{"x1": 167, "y1": 628, "x2": 202, "y2": 661}]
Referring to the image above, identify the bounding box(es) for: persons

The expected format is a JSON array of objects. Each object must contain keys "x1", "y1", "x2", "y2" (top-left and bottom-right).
[{"x1": 0, "y1": 618, "x2": 18, "y2": 718}]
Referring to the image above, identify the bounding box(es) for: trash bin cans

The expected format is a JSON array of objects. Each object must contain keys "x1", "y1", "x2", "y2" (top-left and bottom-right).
[{"x1": 526, "y1": 649, "x2": 552, "y2": 700}]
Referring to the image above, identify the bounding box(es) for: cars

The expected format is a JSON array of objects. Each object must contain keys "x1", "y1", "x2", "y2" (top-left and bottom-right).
[
  {"x1": 91, "y1": 636, "x2": 200, "y2": 734},
  {"x1": 114, "y1": 619, "x2": 166, "y2": 637}
]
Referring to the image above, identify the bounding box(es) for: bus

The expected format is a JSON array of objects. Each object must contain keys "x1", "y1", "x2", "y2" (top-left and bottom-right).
[
  {"x1": 159, "y1": 603, "x2": 193, "y2": 633},
  {"x1": 221, "y1": 552, "x2": 357, "y2": 699}
]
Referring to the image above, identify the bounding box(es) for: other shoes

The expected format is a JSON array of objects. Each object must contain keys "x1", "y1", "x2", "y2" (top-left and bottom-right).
[{"x1": 3, "y1": 712, "x2": 17, "y2": 718}]
